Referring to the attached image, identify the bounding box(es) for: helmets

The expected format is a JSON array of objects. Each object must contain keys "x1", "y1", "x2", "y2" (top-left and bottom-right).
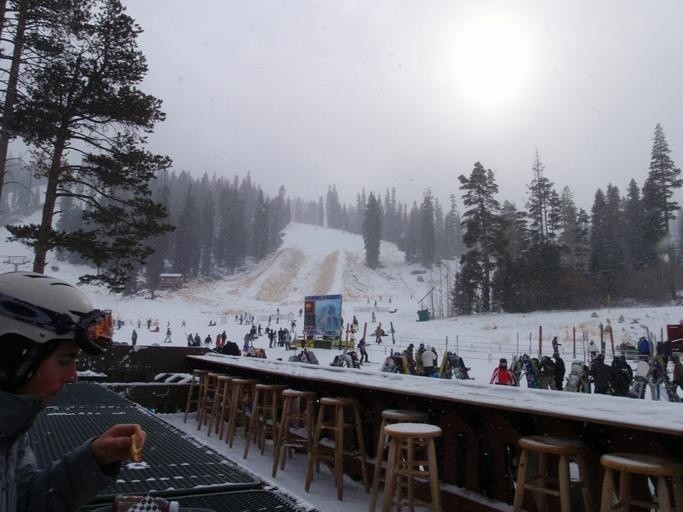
[
  {"x1": 499, "y1": 359, "x2": 507, "y2": 368},
  {"x1": 0, "y1": 270, "x2": 94, "y2": 344}
]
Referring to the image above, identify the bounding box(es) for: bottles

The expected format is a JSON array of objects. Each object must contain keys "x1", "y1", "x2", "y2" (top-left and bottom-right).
[{"x1": 112, "y1": 495, "x2": 178, "y2": 511}]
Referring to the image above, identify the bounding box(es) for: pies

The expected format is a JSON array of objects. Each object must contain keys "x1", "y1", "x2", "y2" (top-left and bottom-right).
[{"x1": 129, "y1": 434, "x2": 144, "y2": 463}]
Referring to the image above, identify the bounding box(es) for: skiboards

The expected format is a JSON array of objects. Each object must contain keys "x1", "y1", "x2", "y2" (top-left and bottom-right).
[{"x1": 653, "y1": 359, "x2": 680, "y2": 401}]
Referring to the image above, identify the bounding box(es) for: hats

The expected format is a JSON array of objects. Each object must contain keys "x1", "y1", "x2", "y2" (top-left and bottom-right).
[{"x1": 409, "y1": 344, "x2": 414, "y2": 347}]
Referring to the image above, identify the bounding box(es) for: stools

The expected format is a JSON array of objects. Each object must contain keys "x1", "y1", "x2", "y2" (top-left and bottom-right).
[
  {"x1": 184, "y1": 368, "x2": 442, "y2": 512},
  {"x1": 600, "y1": 453, "x2": 683, "y2": 512},
  {"x1": 513, "y1": 435, "x2": 595, "y2": 512}
]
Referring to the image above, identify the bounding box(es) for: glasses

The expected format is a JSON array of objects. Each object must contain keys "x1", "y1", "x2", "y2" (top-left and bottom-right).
[{"x1": 1, "y1": 292, "x2": 114, "y2": 357}]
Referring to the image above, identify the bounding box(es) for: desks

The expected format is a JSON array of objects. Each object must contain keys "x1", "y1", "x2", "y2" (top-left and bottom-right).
[{"x1": 189, "y1": 352, "x2": 683, "y2": 511}]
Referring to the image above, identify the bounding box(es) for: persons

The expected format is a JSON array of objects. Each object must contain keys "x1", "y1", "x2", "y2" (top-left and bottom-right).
[
  {"x1": 394, "y1": 343, "x2": 437, "y2": 377},
  {"x1": 1, "y1": 272, "x2": 148, "y2": 512},
  {"x1": 113, "y1": 318, "x2": 171, "y2": 345},
  {"x1": 576, "y1": 336, "x2": 682, "y2": 402},
  {"x1": 489, "y1": 337, "x2": 565, "y2": 390},
  {"x1": 181, "y1": 308, "x2": 386, "y2": 366}
]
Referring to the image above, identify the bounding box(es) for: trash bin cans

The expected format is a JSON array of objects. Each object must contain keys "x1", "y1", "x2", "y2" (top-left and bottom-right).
[{"x1": 418, "y1": 310, "x2": 429, "y2": 321}]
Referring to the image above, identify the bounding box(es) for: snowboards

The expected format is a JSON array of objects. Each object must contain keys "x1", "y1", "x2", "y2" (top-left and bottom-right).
[{"x1": 630, "y1": 361, "x2": 650, "y2": 398}]
